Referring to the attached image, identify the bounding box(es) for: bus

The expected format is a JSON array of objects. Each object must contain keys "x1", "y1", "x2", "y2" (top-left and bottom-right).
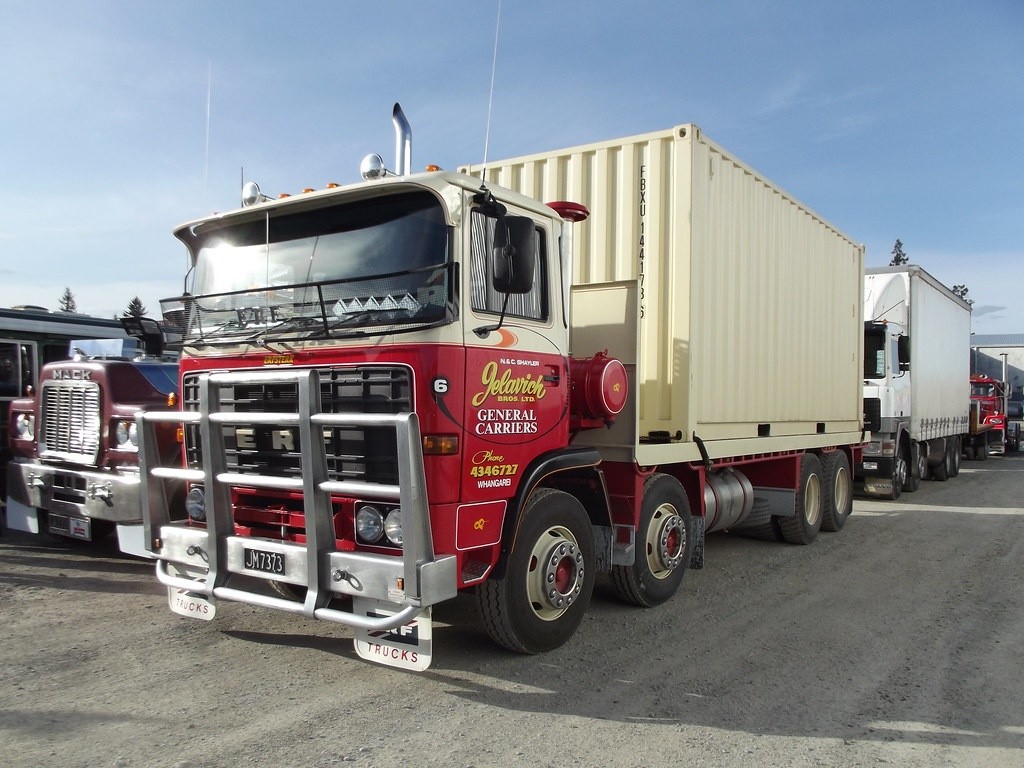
[{"x1": 0, "y1": 305, "x2": 169, "y2": 461}]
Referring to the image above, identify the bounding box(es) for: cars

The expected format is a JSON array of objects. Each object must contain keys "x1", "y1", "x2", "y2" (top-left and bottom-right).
[{"x1": 1008, "y1": 401, "x2": 1024, "y2": 447}]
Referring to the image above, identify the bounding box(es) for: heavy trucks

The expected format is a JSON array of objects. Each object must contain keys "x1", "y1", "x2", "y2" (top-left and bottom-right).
[
  {"x1": 154, "y1": 122, "x2": 890, "y2": 677},
  {"x1": 970, "y1": 373, "x2": 1020, "y2": 460},
  {"x1": 865, "y1": 264, "x2": 974, "y2": 501}
]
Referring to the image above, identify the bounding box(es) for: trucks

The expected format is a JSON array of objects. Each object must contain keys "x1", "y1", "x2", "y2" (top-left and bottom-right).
[{"x1": 5, "y1": 335, "x2": 186, "y2": 564}]
